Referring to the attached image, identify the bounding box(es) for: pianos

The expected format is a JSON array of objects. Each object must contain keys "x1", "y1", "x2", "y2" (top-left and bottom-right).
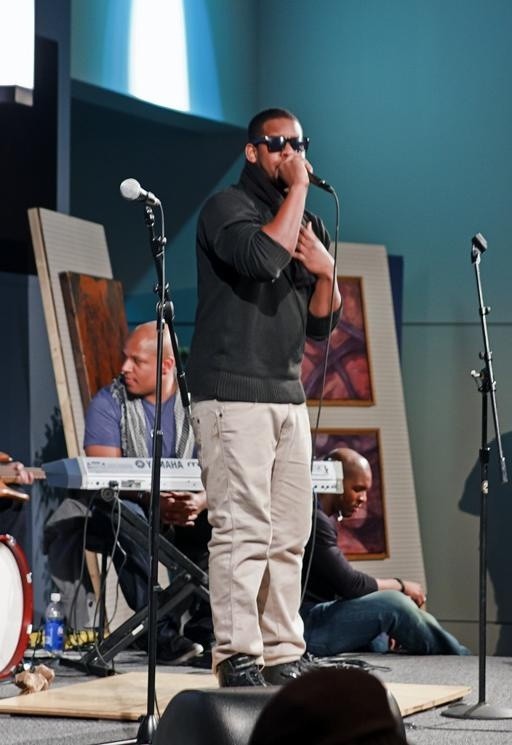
[{"x1": 40, "y1": 455, "x2": 344, "y2": 496}]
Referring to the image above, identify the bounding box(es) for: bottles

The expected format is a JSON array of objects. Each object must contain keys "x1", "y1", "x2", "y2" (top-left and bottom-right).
[{"x1": 43, "y1": 593, "x2": 66, "y2": 659}]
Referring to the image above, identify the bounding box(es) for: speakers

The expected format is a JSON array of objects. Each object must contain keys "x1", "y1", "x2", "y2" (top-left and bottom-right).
[{"x1": 151, "y1": 684, "x2": 286, "y2": 745}]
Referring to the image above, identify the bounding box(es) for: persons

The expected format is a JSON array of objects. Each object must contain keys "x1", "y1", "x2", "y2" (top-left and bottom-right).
[
  {"x1": 458, "y1": 430, "x2": 511, "y2": 657},
  {"x1": 299, "y1": 447, "x2": 471, "y2": 658},
  {"x1": 83, "y1": 319, "x2": 216, "y2": 668},
  {"x1": 185, "y1": 107, "x2": 344, "y2": 688},
  {"x1": 1, "y1": 462, "x2": 35, "y2": 487}
]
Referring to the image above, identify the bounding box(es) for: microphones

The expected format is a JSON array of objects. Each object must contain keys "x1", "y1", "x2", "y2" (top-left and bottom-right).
[
  {"x1": 307, "y1": 169, "x2": 333, "y2": 192},
  {"x1": 120, "y1": 176, "x2": 161, "y2": 207}
]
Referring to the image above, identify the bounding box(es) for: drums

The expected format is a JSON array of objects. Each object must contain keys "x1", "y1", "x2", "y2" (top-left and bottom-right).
[{"x1": 0, "y1": 532, "x2": 35, "y2": 679}]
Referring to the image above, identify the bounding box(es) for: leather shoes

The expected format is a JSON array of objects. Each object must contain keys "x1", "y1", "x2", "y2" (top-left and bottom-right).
[
  {"x1": 261, "y1": 660, "x2": 310, "y2": 685},
  {"x1": 153, "y1": 628, "x2": 205, "y2": 666},
  {"x1": 216, "y1": 652, "x2": 274, "y2": 687}
]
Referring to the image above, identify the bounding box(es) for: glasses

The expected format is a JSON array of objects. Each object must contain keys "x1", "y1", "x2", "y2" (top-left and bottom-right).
[{"x1": 251, "y1": 133, "x2": 309, "y2": 152}]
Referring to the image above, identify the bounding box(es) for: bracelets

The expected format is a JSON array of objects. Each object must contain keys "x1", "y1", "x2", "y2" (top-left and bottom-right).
[{"x1": 394, "y1": 575, "x2": 405, "y2": 592}]
